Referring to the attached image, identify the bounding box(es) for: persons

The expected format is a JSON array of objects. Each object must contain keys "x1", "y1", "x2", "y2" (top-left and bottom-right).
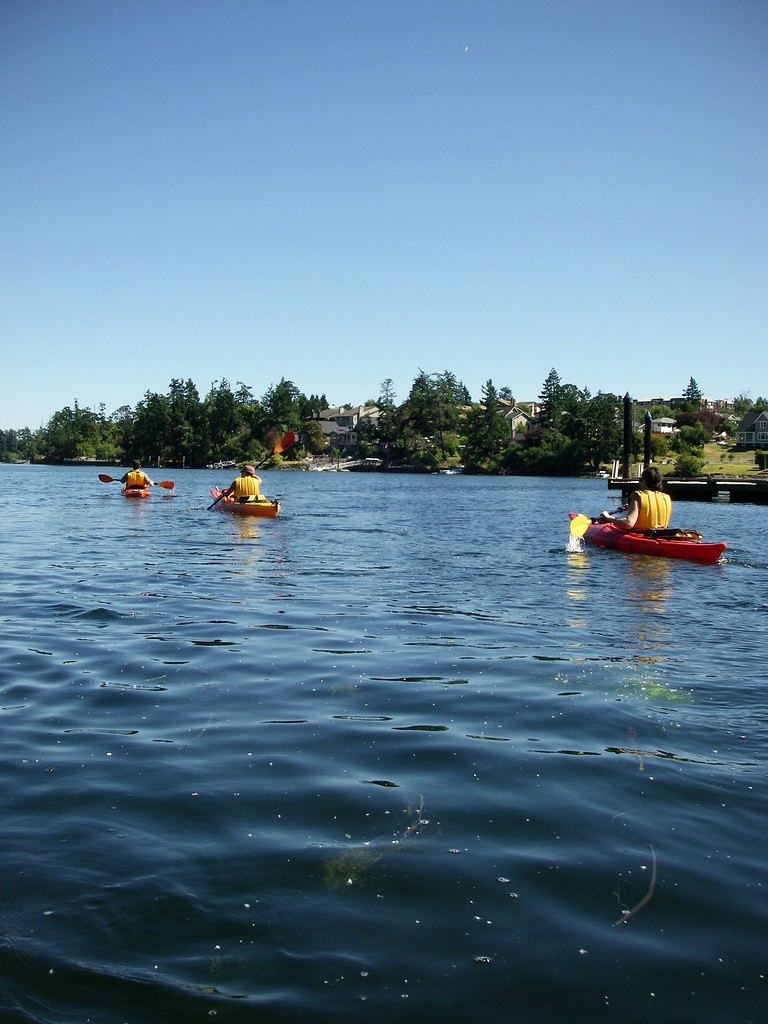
[
  {"x1": 601, "y1": 467, "x2": 672, "y2": 533},
  {"x1": 222, "y1": 465, "x2": 262, "y2": 502},
  {"x1": 121, "y1": 459, "x2": 154, "y2": 488}
]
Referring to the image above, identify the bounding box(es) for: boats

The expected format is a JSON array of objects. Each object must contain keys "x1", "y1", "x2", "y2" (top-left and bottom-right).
[
  {"x1": 210, "y1": 485, "x2": 281, "y2": 518},
  {"x1": 120, "y1": 486, "x2": 151, "y2": 500},
  {"x1": 568, "y1": 511, "x2": 727, "y2": 567}
]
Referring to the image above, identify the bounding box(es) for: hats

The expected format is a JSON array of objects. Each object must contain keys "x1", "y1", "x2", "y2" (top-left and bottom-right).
[
  {"x1": 645, "y1": 467, "x2": 662, "y2": 490},
  {"x1": 240, "y1": 465, "x2": 255, "y2": 474}
]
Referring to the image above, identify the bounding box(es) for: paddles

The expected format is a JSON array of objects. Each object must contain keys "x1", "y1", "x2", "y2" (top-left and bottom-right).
[
  {"x1": 97, "y1": 473, "x2": 174, "y2": 490},
  {"x1": 569, "y1": 499, "x2": 630, "y2": 538},
  {"x1": 206, "y1": 430, "x2": 296, "y2": 511}
]
